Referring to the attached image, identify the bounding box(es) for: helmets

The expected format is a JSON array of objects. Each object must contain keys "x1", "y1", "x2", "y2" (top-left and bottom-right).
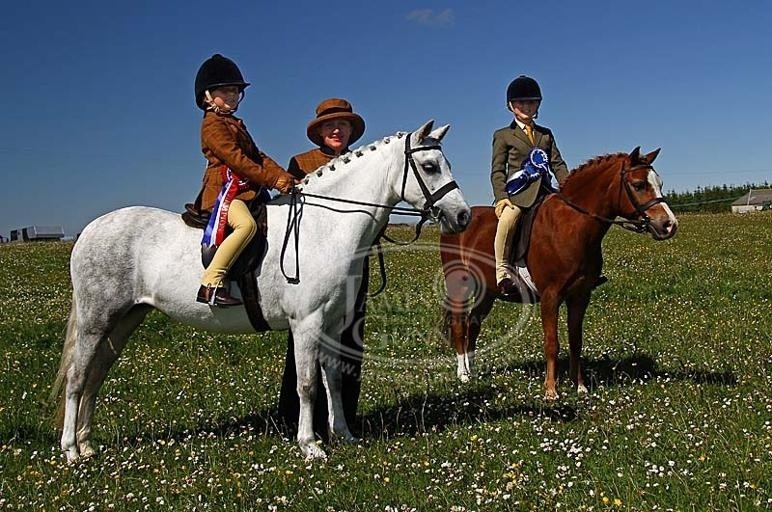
[
  {"x1": 195, "y1": 54, "x2": 250, "y2": 108},
  {"x1": 507, "y1": 75, "x2": 541, "y2": 103}
]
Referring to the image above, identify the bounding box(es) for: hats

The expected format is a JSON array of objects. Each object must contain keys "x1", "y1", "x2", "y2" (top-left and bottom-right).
[{"x1": 306, "y1": 99, "x2": 365, "y2": 146}]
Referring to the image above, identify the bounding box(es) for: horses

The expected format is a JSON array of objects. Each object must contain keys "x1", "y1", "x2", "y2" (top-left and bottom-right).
[
  {"x1": 434, "y1": 144, "x2": 680, "y2": 403},
  {"x1": 44, "y1": 118, "x2": 472, "y2": 470}
]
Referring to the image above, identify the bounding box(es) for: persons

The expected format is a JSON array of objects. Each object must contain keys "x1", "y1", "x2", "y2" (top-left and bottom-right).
[
  {"x1": 490, "y1": 75, "x2": 569, "y2": 301},
  {"x1": 276, "y1": 97, "x2": 369, "y2": 443},
  {"x1": 194, "y1": 51, "x2": 301, "y2": 307}
]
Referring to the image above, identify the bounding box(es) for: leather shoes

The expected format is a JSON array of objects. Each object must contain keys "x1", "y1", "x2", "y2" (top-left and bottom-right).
[
  {"x1": 497, "y1": 278, "x2": 518, "y2": 299},
  {"x1": 197, "y1": 285, "x2": 243, "y2": 306}
]
